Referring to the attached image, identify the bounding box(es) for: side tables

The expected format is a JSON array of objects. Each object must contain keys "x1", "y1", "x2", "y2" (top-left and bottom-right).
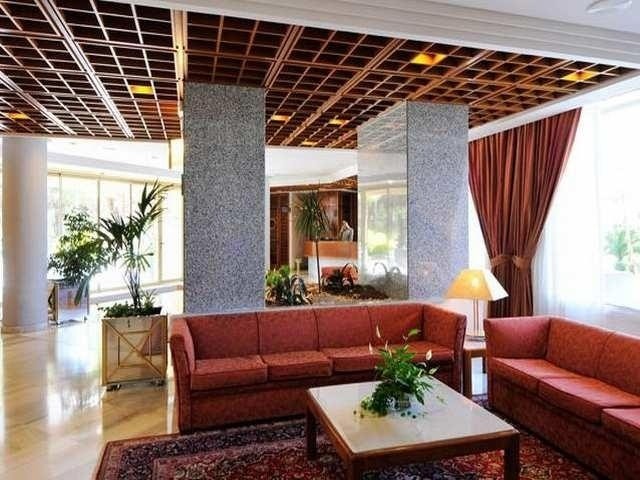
[{"x1": 460, "y1": 336, "x2": 487, "y2": 397}]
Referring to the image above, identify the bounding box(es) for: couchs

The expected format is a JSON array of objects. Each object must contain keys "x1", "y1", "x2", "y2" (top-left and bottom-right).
[
  {"x1": 166, "y1": 300, "x2": 468, "y2": 441},
  {"x1": 480, "y1": 312, "x2": 640, "y2": 480}
]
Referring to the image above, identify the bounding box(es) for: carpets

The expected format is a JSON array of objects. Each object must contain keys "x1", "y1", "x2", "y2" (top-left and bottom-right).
[{"x1": 84, "y1": 408, "x2": 603, "y2": 480}]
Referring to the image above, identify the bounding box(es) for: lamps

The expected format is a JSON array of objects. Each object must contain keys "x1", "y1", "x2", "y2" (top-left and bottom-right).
[{"x1": 444, "y1": 267, "x2": 512, "y2": 340}]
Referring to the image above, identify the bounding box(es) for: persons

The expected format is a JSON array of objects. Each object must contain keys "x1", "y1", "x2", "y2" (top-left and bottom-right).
[{"x1": 339, "y1": 220, "x2": 354, "y2": 241}]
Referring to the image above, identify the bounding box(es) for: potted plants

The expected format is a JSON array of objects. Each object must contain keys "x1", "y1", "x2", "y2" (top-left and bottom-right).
[
  {"x1": 45, "y1": 172, "x2": 180, "y2": 390},
  {"x1": 46, "y1": 200, "x2": 128, "y2": 324},
  {"x1": 353, "y1": 318, "x2": 448, "y2": 423}
]
